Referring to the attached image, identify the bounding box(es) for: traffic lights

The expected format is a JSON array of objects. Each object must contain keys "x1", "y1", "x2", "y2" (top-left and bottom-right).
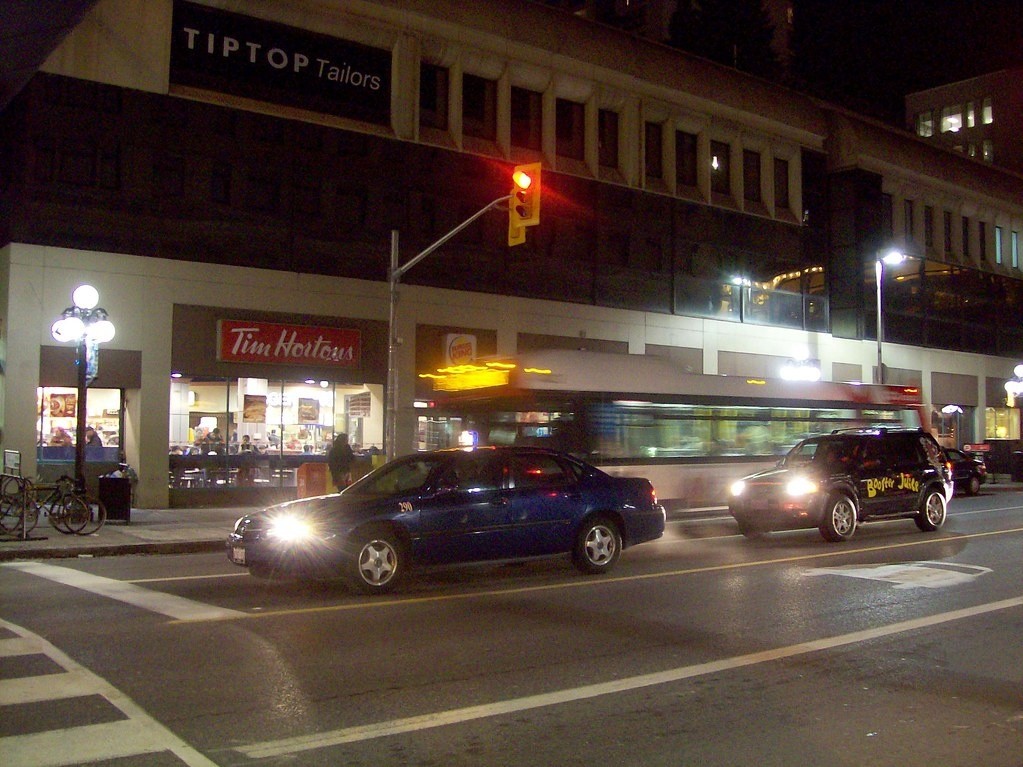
[{"x1": 514, "y1": 161, "x2": 540, "y2": 227}]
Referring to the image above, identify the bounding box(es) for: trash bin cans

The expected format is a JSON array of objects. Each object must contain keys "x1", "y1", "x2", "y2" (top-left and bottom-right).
[
  {"x1": 97, "y1": 477, "x2": 132, "y2": 525},
  {"x1": 1010, "y1": 450, "x2": 1023, "y2": 482}
]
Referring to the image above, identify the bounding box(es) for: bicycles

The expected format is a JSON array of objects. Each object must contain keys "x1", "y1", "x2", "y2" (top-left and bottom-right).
[{"x1": 0, "y1": 472, "x2": 107, "y2": 537}]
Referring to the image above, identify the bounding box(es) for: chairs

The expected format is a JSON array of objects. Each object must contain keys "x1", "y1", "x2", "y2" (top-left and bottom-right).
[{"x1": 170, "y1": 445, "x2": 296, "y2": 489}]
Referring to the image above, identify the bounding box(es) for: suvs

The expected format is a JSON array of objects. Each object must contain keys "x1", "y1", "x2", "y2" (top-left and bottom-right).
[{"x1": 727, "y1": 425, "x2": 952, "y2": 542}]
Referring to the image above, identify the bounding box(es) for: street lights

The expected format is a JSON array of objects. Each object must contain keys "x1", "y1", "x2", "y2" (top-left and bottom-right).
[
  {"x1": 1003, "y1": 364, "x2": 1023, "y2": 451},
  {"x1": 50, "y1": 283, "x2": 116, "y2": 525},
  {"x1": 875, "y1": 252, "x2": 903, "y2": 381}
]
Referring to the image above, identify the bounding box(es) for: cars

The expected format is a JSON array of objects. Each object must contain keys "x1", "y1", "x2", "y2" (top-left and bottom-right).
[
  {"x1": 942, "y1": 450, "x2": 988, "y2": 495},
  {"x1": 226, "y1": 444, "x2": 664, "y2": 594}
]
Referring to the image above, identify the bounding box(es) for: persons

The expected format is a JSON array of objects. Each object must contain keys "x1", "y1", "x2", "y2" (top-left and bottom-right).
[
  {"x1": 266, "y1": 426, "x2": 339, "y2": 456},
  {"x1": 50, "y1": 426, "x2": 73, "y2": 447},
  {"x1": 170, "y1": 427, "x2": 262, "y2": 455},
  {"x1": 85, "y1": 420, "x2": 104, "y2": 447},
  {"x1": 328, "y1": 434, "x2": 355, "y2": 493}
]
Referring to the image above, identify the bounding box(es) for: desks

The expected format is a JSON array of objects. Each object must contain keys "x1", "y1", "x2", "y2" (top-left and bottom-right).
[
  {"x1": 264, "y1": 454, "x2": 369, "y2": 488},
  {"x1": 170, "y1": 454, "x2": 246, "y2": 488}
]
